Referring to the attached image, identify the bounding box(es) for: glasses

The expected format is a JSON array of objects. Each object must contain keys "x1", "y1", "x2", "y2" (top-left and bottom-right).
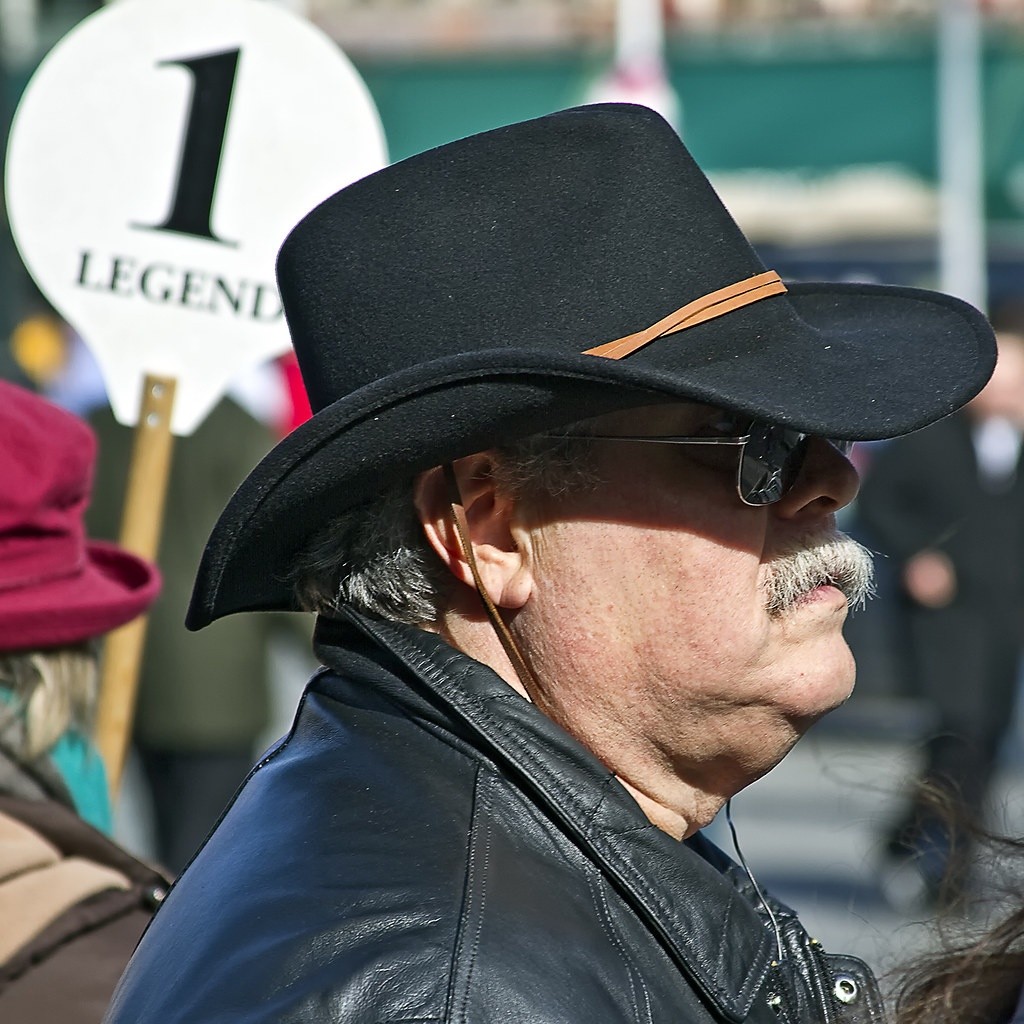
[{"x1": 519, "y1": 416, "x2": 855, "y2": 506}]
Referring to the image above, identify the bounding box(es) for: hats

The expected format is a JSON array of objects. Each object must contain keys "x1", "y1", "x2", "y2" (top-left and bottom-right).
[
  {"x1": 184, "y1": 103, "x2": 998, "y2": 631},
  {"x1": 0, "y1": 380, "x2": 162, "y2": 647}
]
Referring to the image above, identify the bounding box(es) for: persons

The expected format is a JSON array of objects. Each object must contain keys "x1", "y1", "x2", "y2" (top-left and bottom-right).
[
  {"x1": 0, "y1": 325, "x2": 1024, "y2": 1024},
  {"x1": 106, "y1": 104, "x2": 999, "y2": 1024}
]
